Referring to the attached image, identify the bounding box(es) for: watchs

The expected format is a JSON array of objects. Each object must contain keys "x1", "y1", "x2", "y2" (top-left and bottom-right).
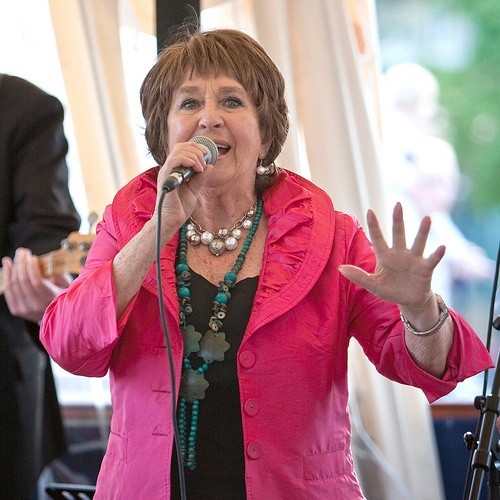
[{"x1": 400, "y1": 293, "x2": 450, "y2": 336}]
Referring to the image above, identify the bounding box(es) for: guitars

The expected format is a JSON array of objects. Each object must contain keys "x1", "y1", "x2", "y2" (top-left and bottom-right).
[{"x1": 0, "y1": 212, "x2": 98, "y2": 294}]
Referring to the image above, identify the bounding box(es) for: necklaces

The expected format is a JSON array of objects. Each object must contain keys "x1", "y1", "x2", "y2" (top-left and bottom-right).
[
  {"x1": 177, "y1": 199, "x2": 263, "y2": 471},
  {"x1": 186, "y1": 201, "x2": 258, "y2": 257}
]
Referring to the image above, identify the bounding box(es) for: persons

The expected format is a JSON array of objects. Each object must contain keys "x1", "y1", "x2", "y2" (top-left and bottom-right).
[
  {"x1": 0, "y1": 74, "x2": 81, "y2": 500},
  {"x1": 39, "y1": 29, "x2": 496, "y2": 500}
]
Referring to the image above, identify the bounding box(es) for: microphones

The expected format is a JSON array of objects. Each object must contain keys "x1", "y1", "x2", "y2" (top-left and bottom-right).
[{"x1": 161, "y1": 135, "x2": 220, "y2": 193}]
